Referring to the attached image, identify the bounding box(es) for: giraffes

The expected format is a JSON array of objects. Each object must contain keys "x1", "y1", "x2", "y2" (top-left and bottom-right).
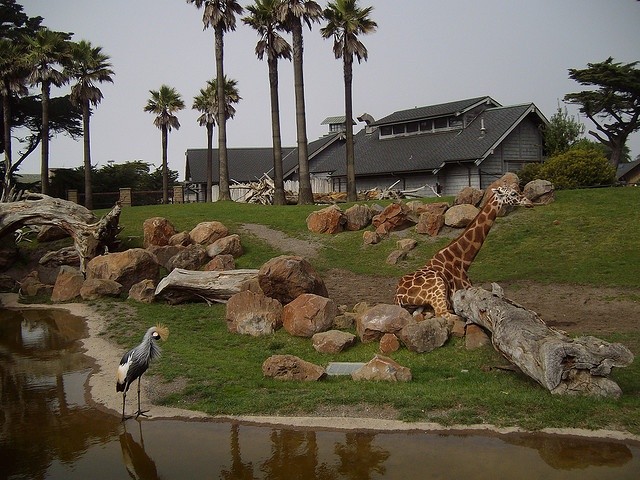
[{"x1": 396, "y1": 179, "x2": 533, "y2": 319}]
